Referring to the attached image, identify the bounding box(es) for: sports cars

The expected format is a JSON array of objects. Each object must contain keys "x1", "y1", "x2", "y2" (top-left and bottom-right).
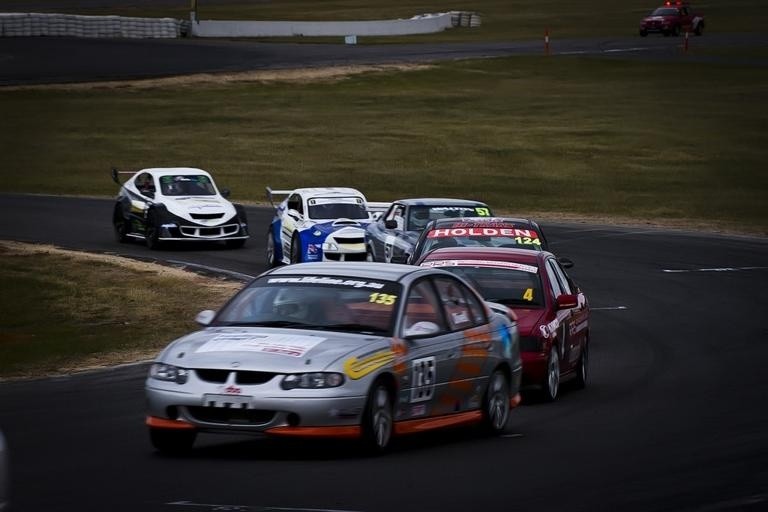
[
  {"x1": 110, "y1": 166, "x2": 252, "y2": 252},
  {"x1": 264, "y1": 185, "x2": 373, "y2": 268}
]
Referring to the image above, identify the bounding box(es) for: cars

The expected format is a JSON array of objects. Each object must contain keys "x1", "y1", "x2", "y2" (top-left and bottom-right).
[
  {"x1": 413, "y1": 246, "x2": 590, "y2": 400},
  {"x1": 406, "y1": 216, "x2": 576, "y2": 270},
  {"x1": 364, "y1": 198, "x2": 496, "y2": 263},
  {"x1": 142, "y1": 260, "x2": 522, "y2": 462}
]
